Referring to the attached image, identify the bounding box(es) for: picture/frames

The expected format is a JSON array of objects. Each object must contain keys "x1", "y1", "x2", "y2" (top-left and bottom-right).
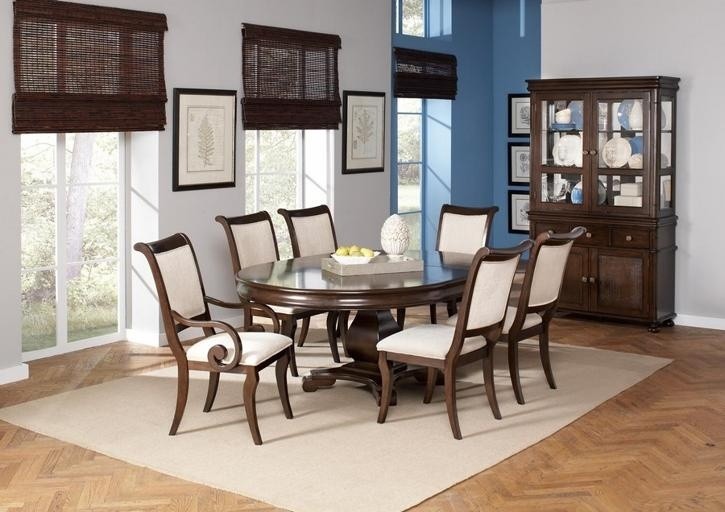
[
  {"x1": 506, "y1": 93, "x2": 533, "y2": 236},
  {"x1": 342, "y1": 88, "x2": 385, "y2": 176},
  {"x1": 172, "y1": 88, "x2": 236, "y2": 193}
]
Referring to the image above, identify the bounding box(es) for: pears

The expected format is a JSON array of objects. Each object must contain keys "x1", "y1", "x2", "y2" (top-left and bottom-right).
[{"x1": 335, "y1": 245, "x2": 374, "y2": 257}]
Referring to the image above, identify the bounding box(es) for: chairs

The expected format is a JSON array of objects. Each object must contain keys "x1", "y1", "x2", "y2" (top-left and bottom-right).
[
  {"x1": 215, "y1": 210, "x2": 339, "y2": 378},
  {"x1": 275, "y1": 205, "x2": 338, "y2": 363},
  {"x1": 133, "y1": 233, "x2": 295, "y2": 443},
  {"x1": 430, "y1": 203, "x2": 501, "y2": 324},
  {"x1": 448, "y1": 224, "x2": 588, "y2": 404},
  {"x1": 375, "y1": 239, "x2": 534, "y2": 440}
]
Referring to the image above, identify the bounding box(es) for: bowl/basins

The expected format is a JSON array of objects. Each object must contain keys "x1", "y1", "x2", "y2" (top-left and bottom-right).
[{"x1": 330, "y1": 251, "x2": 381, "y2": 266}]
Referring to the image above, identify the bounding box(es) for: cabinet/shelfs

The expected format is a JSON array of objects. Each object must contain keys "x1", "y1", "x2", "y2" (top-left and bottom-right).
[{"x1": 524, "y1": 76, "x2": 681, "y2": 333}]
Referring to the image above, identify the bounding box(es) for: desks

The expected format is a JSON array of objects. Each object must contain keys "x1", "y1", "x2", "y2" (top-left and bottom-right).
[{"x1": 235, "y1": 250, "x2": 478, "y2": 406}]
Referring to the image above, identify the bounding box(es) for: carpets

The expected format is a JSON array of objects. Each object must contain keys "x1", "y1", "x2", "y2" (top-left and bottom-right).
[{"x1": 2, "y1": 333, "x2": 676, "y2": 512}]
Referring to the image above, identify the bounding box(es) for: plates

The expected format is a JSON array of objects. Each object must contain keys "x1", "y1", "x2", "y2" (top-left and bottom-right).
[{"x1": 547, "y1": 100, "x2": 669, "y2": 209}]
